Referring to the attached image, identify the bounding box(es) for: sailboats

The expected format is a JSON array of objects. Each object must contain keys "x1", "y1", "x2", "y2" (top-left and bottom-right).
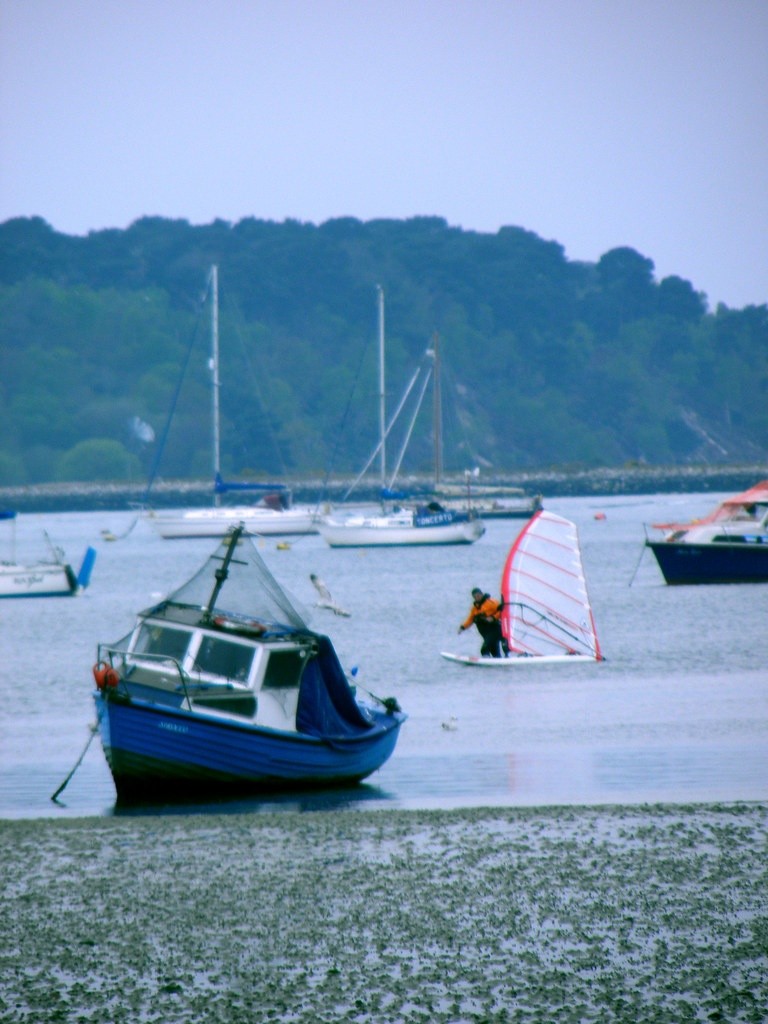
[
  {"x1": 436, "y1": 508, "x2": 609, "y2": 669},
  {"x1": 93, "y1": 517, "x2": 410, "y2": 799},
  {"x1": 148, "y1": 262, "x2": 339, "y2": 538},
  {"x1": 306, "y1": 323, "x2": 488, "y2": 549},
  {"x1": 385, "y1": 332, "x2": 546, "y2": 525}
]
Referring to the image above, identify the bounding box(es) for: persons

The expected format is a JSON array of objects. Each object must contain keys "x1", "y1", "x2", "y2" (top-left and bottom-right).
[{"x1": 459, "y1": 588, "x2": 502, "y2": 658}]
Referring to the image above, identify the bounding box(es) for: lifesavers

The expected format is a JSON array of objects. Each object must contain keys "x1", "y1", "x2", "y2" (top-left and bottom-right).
[{"x1": 214, "y1": 615, "x2": 267, "y2": 636}]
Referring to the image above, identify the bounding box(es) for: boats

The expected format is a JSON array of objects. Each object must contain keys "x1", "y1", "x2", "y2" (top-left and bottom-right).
[
  {"x1": 0, "y1": 524, "x2": 101, "y2": 599},
  {"x1": 642, "y1": 475, "x2": 766, "y2": 587}
]
